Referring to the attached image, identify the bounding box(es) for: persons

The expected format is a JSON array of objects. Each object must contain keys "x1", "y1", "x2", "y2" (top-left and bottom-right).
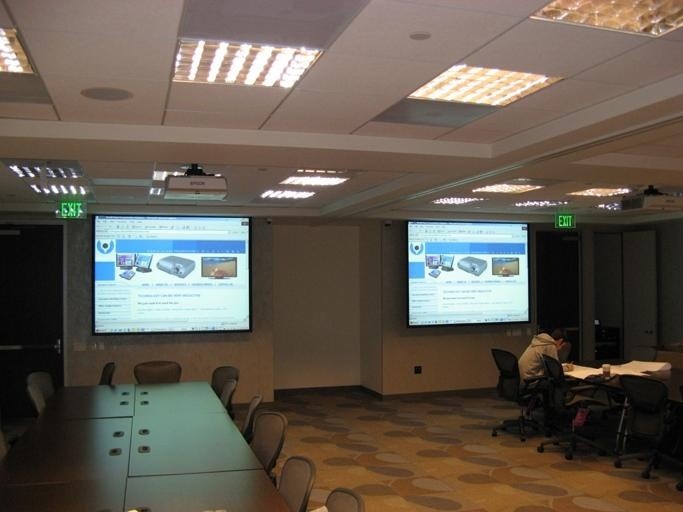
[{"x1": 516, "y1": 327, "x2": 568, "y2": 428}]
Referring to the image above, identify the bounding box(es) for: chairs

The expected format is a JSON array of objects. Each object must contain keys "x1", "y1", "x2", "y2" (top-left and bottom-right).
[
  {"x1": 488, "y1": 346, "x2": 683, "y2": 493},
  {"x1": 210, "y1": 365, "x2": 241, "y2": 419},
  {"x1": 276, "y1": 454, "x2": 318, "y2": 510},
  {"x1": 27, "y1": 383, "x2": 45, "y2": 414},
  {"x1": 242, "y1": 395, "x2": 265, "y2": 440},
  {"x1": 218, "y1": 377, "x2": 237, "y2": 408},
  {"x1": 322, "y1": 484, "x2": 365, "y2": 510},
  {"x1": 25, "y1": 370, "x2": 56, "y2": 399},
  {"x1": 249, "y1": 410, "x2": 290, "y2": 475},
  {"x1": 132, "y1": 361, "x2": 184, "y2": 385},
  {"x1": 96, "y1": 362, "x2": 118, "y2": 385}
]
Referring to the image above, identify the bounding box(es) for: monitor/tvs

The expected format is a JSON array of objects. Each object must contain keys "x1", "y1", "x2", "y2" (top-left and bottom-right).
[
  {"x1": 201, "y1": 257, "x2": 238, "y2": 277},
  {"x1": 116, "y1": 253, "x2": 152, "y2": 271},
  {"x1": 492, "y1": 256, "x2": 520, "y2": 277},
  {"x1": 426, "y1": 255, "x2": 454, "y2": 270}
]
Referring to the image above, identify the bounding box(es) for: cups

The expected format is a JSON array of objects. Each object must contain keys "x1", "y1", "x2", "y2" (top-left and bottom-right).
[{"x1": 602, "y1": 364, "x2": 610, "y2": 380}]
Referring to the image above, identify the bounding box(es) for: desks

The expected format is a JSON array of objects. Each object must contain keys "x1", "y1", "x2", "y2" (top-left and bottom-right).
[
  {"x1": 2, "y1": 417, "x2": 132, "y2": 487},
  {"x1": 128, "y1": 380, "x2": 268, "y2": 475},
  {"x1": 34, "y1": 381, "x2": 137, "y2": 420},
  {"x1": 121, "y1": 468, "x2": 293, "y2": 510},
  {"x1": 2, "y1": 479, "x2": 128, "y2": 508}
]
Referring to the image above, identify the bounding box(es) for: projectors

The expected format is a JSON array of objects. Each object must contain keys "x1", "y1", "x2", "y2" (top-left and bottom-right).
[
  {"x1": 458, "y1": 256, "x2": 486, "y2": 276},
  {"x1": 158, "y1": 256, "x2": 195, "y2": 278},
  {"x1": 620, "y1": 193, "x2": 683, "y2": 213},
  {"x1": 162, "y1": 172, "x2": 230, "y2": 202}
]
